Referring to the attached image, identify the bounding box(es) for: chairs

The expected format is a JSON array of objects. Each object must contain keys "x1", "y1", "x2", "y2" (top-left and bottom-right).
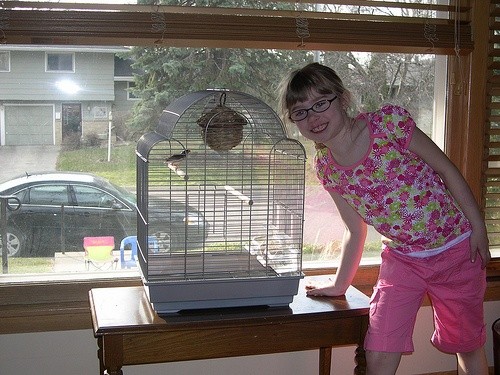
[
  {"x1": 83, "y1": 236, "x2": 119, "y2": 272},
  {"x1": 121, "y1": 235, "x2": 158, "y2": 269}
]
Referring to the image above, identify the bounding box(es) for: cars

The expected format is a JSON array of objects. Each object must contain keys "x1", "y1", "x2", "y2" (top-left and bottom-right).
[{"x1": 0, "y1": 171, "x2": 210, "y2": 257}]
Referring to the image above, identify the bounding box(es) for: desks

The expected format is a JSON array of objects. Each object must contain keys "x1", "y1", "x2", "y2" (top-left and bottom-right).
[{"x1": 89, "y1": 274, "x2": 370, "y2": 375}]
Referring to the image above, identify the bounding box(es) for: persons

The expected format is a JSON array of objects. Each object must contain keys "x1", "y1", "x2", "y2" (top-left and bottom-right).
[{"x1": 276, "y1": 62, "x2": 491, "y2": 375}]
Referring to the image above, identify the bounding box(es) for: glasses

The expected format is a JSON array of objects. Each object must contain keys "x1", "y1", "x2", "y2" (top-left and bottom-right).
[{"x1": 288, "y1": 96, "x2": 338, "y2": 124}]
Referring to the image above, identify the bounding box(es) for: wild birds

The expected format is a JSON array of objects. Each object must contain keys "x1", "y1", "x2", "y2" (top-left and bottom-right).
[{"x1": 164, "y1": 149, "x2": 191, "y2": 172}]
